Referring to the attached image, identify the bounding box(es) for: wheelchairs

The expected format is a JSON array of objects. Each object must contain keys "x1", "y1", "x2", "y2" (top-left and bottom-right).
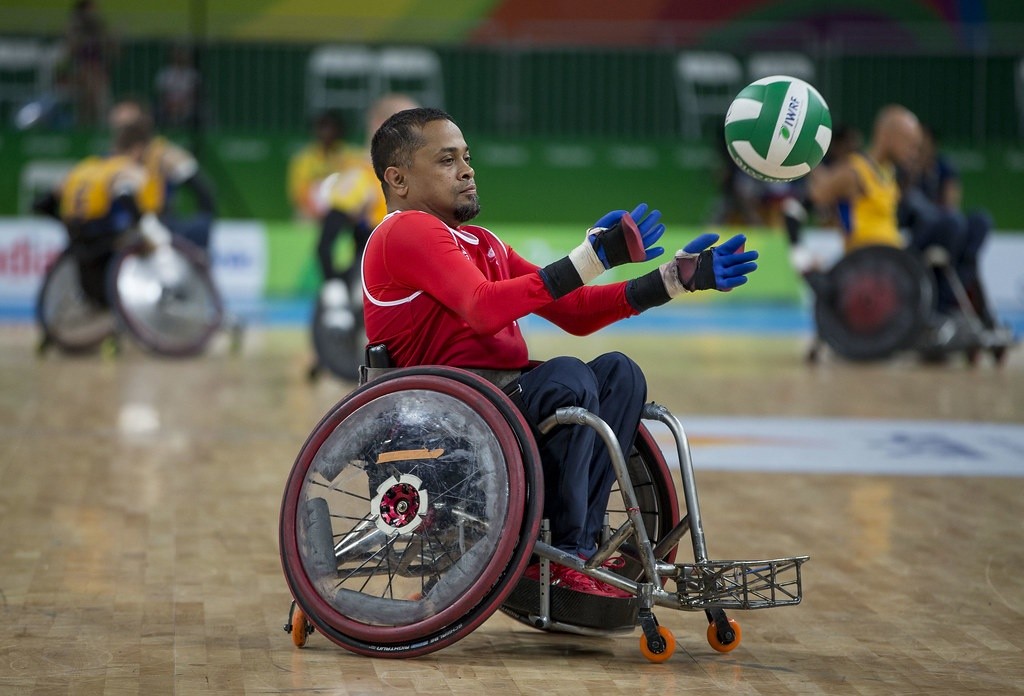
[
  {"x1": 37, "y1": 240, "x2": 227, "y2": 360},
  {"x1": 808, "y1": 244, "x2": 1018, "y2": 368},
  {"x1": 275, "y1": 342, "x2": 812, "y2": 663}
]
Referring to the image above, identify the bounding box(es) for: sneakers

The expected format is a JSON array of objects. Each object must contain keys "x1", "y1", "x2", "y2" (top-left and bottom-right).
[{"x1": 523, "y1": 553, "x2": 635, "y2": 599}]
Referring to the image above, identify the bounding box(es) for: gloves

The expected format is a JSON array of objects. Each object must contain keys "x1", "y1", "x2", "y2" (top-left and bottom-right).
[
  {"x1": 538, "y1": 203, "x2": 666, "y2": 300},
  {"x1": 623, "y1": 233, "x2": 759, "y2": 314}
]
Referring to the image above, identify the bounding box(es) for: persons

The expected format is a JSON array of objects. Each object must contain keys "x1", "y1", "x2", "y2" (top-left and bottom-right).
[
  {"x1": 287, "y1": 105, "x2": 411, "y2": 278},
  {"x1": 778, "y1": 102, "x2": 993, "y2": 354},
  {"x1": 363, "y1": 100, "x2": 764, "y2": 623},
  {"x1": 58, "y1": 100, "x2": 222, "y2": 312}
]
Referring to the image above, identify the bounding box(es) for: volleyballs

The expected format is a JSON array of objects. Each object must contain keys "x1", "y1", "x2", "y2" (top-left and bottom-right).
[{"x1": 725, "y1": 74, "x2": 835, "y2": 184}]
